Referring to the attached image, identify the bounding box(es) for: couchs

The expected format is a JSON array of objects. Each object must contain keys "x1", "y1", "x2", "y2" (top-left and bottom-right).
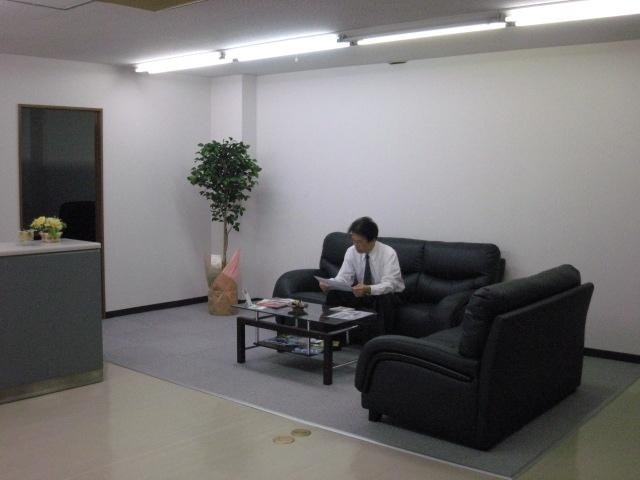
[
  {"x1": 354, "y1": 262, "x2": 594, "y2": 453},
  {"x1": 272, "y1": 231, "x2": 507, "y2": 353}
]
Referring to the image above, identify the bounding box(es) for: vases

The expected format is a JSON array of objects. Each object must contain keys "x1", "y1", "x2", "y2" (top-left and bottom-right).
[{"x1": 37, "y1": 231, "x2": 64, "y2": 242}]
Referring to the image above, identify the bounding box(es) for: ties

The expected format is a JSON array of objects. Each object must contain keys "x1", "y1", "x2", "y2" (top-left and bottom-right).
[{"x1": 363, "y1": 256, "x2": 373, "y2": 286}]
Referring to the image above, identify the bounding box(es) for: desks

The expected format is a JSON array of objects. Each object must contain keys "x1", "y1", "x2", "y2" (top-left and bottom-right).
[{"x1": 0, "y1": 238, "x2": 104, "y2": 406}]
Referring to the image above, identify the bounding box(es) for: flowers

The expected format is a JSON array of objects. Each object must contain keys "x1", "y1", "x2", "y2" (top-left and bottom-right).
[{"x1": 30, "y1": 215, "x2": 67, "y2": 238}]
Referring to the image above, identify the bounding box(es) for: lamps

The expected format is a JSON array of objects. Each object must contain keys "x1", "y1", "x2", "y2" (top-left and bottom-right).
[
  {"x1": 134, "y1": 50, "x2": 235, "y2": 75},
  {"x1": 507, "y1": 1, "x2": 638, "y2": 27},
  {"x1": 342, "y1": 13, "x2": 507, "y2": 47},
  {"x1": 221, "y1": 33, "x2": 356, "y2": 64}
]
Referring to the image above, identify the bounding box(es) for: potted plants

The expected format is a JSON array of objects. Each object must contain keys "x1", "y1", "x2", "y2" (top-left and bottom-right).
[{"x1": 186, "y1": 135, "x2": 262, "y2": 315}]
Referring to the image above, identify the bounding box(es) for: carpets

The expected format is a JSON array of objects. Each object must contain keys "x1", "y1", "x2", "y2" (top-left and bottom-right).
[{"x1": 102, "y1": 300, "x2": 640, "y2": 480}]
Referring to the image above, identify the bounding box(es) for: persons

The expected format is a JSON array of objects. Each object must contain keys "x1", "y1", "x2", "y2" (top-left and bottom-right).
[{"x1": 317, "y1": 216, "x2": 407, "y2": 353}]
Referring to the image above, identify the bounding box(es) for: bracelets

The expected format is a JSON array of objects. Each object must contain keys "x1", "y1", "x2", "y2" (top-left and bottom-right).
[{"x1": 367, "y1": 286, "x2": 371, "y2": 294}]
